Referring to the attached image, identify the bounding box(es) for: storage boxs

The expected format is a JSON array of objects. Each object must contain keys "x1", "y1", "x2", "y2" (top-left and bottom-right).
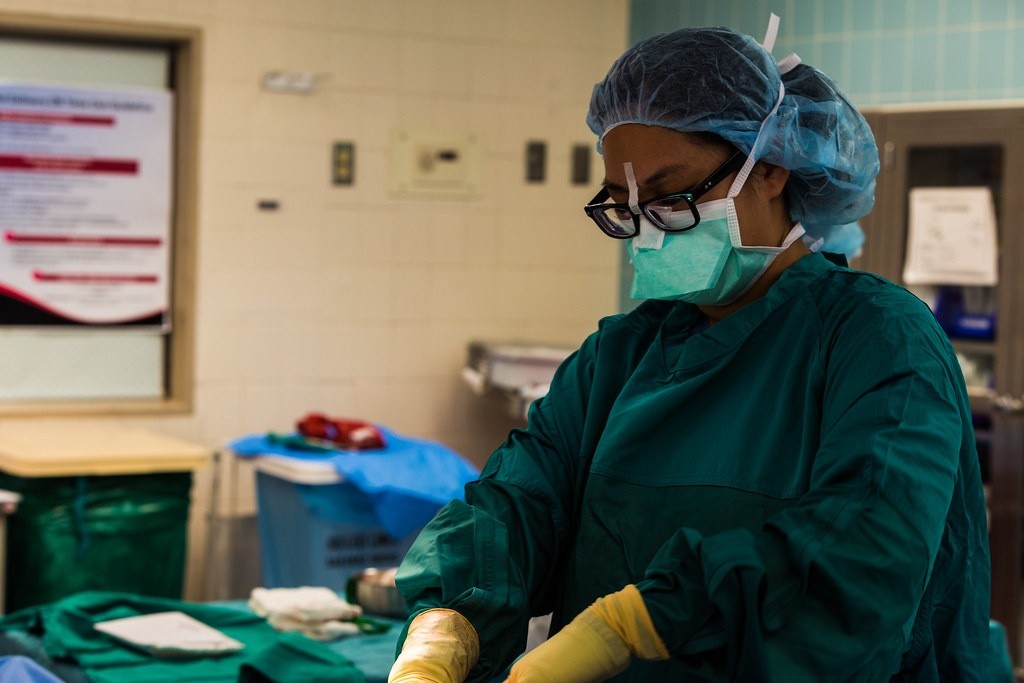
[{"x1": 256, "y1": 455, "x2": 396, "y2": 605}]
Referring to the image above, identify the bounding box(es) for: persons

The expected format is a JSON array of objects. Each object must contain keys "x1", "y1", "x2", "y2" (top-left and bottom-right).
[{"x1": 388, "y1": 28, "x2": 993, "y2": 683}]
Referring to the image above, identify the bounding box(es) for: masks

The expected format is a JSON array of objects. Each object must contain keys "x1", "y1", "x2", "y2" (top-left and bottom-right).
[{"x1": 629, "y1": 154, "x2": 804, "y2": 304}]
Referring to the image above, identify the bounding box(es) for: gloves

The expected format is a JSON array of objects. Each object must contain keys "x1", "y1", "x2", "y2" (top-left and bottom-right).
[
  {"x1": 499, "y1": 583, "x2": 670, "y2": 683},
  {"x1": 387, "y1": 605, "x2": 481, "y2": 683}
]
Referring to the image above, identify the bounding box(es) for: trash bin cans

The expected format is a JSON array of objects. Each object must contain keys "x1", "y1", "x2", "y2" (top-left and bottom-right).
[{"x1": 252, "y1": 454, "x2": 406, "y2": 595}]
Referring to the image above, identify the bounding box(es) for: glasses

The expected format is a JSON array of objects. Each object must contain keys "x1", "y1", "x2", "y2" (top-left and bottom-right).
[{"x1": 584, "y1": 152, "x2": 745, "y2": 240}]
